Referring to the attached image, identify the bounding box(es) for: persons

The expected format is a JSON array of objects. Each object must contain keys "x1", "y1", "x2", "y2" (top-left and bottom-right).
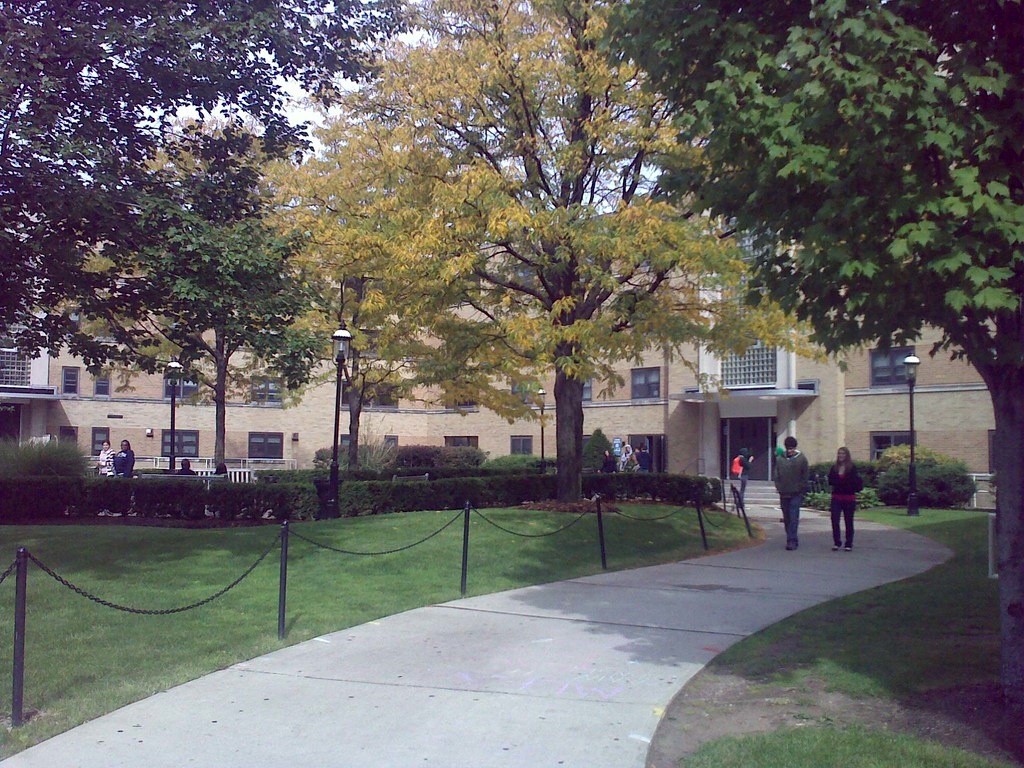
[
  {"x1": 596, "y1": 441, "x2": 651, "y2": 473},
  {"x1": 95, "y1": 441, "x2": 115, "y2": 478},
  {"x1": 213, "y1": 461, "x2": 228, "y2": 479},
  {"x1": 112, "y1": 439, "x2": 135, "y2": 478},
  {"x1": 773, "y1": 436, "x2": 809, "y2": 550},
  {"x1": 173, "y1": 459, "x2": 197, "y2": 475},
  {"x1": 736, "y1": 447, "x2": 754, "y2": 507},
  {"x1": 827, "y1": 447, "x2": 859, "y2": 551}
]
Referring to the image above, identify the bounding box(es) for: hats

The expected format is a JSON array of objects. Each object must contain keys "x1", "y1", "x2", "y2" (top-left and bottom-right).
[{"x1": 739, "y1": 447, "x2": 748, "y2": 454}]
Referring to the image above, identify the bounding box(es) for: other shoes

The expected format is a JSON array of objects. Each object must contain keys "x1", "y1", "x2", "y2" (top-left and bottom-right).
[
  {"x1": 844, "y1": 546, "x2": 852, "y2": 551},
  {"x1": 786, "y1": 539, "x2": 798, "y2": 550},
  {"x1": 832, "y1": 545, "x2": 839, "y2": 551}
]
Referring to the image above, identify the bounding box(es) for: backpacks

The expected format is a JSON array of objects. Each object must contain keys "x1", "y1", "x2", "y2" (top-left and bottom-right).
[{"x1": 730, "y1": 455, "x2": 743, "y2": 475}]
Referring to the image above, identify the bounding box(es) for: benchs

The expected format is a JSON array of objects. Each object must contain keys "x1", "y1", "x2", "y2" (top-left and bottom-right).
[
  {"x1": 136, "y1": 474, "x2": 229, "y2": 483},
  {"x1": 392, "y1": 473, "x2": 431, "y2": 482}
]
